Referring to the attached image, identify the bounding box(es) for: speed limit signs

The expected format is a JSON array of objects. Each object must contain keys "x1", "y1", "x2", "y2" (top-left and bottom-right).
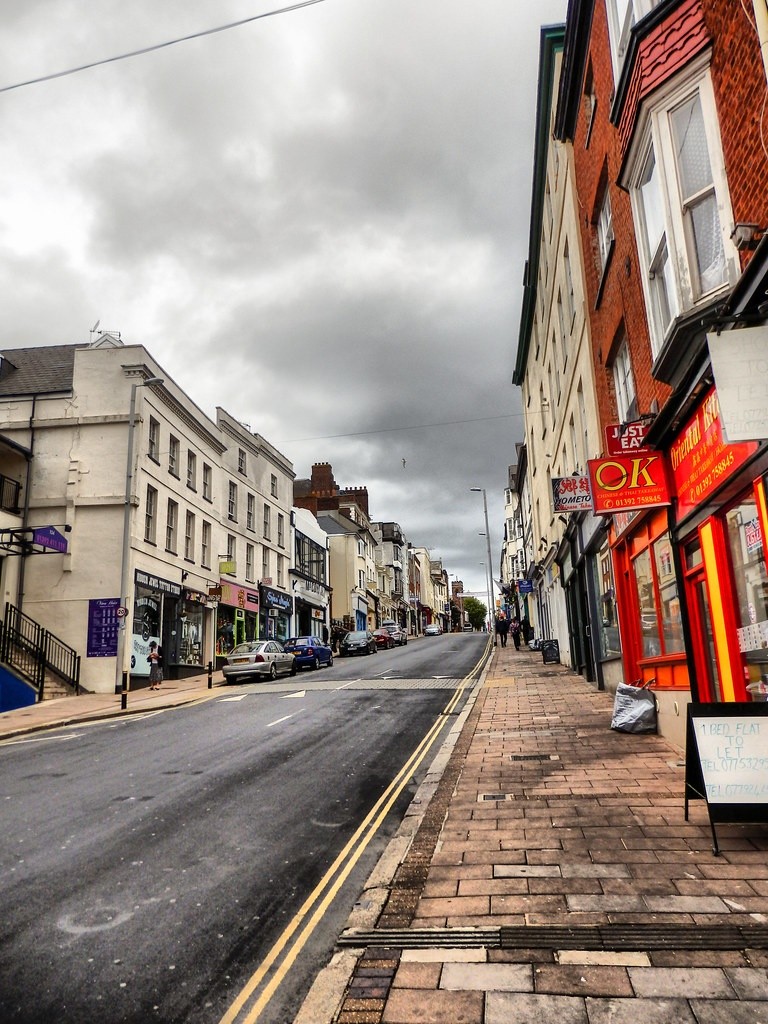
[{"x1": 116, "y1": 608, "x2": 125, "y2": 617}]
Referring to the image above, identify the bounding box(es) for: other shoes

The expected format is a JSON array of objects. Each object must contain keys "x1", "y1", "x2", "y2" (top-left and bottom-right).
[
  {"x1": 155, "y1": 688, "x2": 159, "y2": 690},
  {"x1": 149, "y1": 688, "x2": 153, "y2": 690}
]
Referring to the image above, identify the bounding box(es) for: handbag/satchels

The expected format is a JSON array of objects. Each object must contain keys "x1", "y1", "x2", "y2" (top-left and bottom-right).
[{"x1": 610, "y1": 678, "x2": 656, "y2": 732}]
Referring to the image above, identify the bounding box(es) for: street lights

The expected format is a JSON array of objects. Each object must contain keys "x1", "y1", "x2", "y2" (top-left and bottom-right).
[
  {"x1": 470, "y1": 487, "x2": 497, "y2": 646},
  {"x1": 479, "y1": 561, "x2": 491, "y2": 634},
  {"x1": 412, "y1": 552, "x2": 422, "y2": 637},
  {"x1": 113, "y1": 377, "x2": 164, "y2": 693},
  {"x1": 448, "y1": 574, "x2": 454, "y2": 633}
]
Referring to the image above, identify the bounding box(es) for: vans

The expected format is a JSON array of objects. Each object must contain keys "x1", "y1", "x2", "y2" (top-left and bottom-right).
[
  {"x1": 463, "y1": 623, "x2": 472, "y2": 632},
  {"x1": 642, "y1": 613, "x2": 657, "y2": 637}
]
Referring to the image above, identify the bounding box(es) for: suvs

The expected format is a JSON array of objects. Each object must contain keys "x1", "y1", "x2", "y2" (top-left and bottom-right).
[{"x1": 386, "y1": 626, "x2": 407, "y2": 646}]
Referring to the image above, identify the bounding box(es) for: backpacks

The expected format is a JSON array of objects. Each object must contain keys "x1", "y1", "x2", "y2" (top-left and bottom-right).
[{"x1": 512, "y1": 624, "x2": 520, "y2": 635}]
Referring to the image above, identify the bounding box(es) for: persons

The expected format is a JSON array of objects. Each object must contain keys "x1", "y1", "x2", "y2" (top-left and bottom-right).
[
  {"x1": 148, "y1": 641, "x2": 165, "y2": 690},
  {"x1": 497, "y1": 615, "x2": 532, "y2": 650},
  {"x1": 323, "y1": 623, "x2": 329, "y2": 644}
]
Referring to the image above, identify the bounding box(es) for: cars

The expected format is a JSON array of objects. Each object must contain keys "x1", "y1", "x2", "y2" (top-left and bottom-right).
[
  {"x1": 283, "y1": 636, "x2": 334, "y2": 670},
  {"x1": 602, "y1": 616, "x2": 611, "y2": 627},
  {"x1": 222, "y1": 640, "x2": 297, "y2": 685},
  {"x1": 652, "y1": 617, "x2": 679, "y2": 639},
  {"x1": 424, "y1": 624, "x2": 442, "y2": 636},
  {"x1": 339, "y1": 630, "x2": 378, "y2": 657},
  {"x1": 372, "y1": 629, "x2": 395, "y2": 649}
]
